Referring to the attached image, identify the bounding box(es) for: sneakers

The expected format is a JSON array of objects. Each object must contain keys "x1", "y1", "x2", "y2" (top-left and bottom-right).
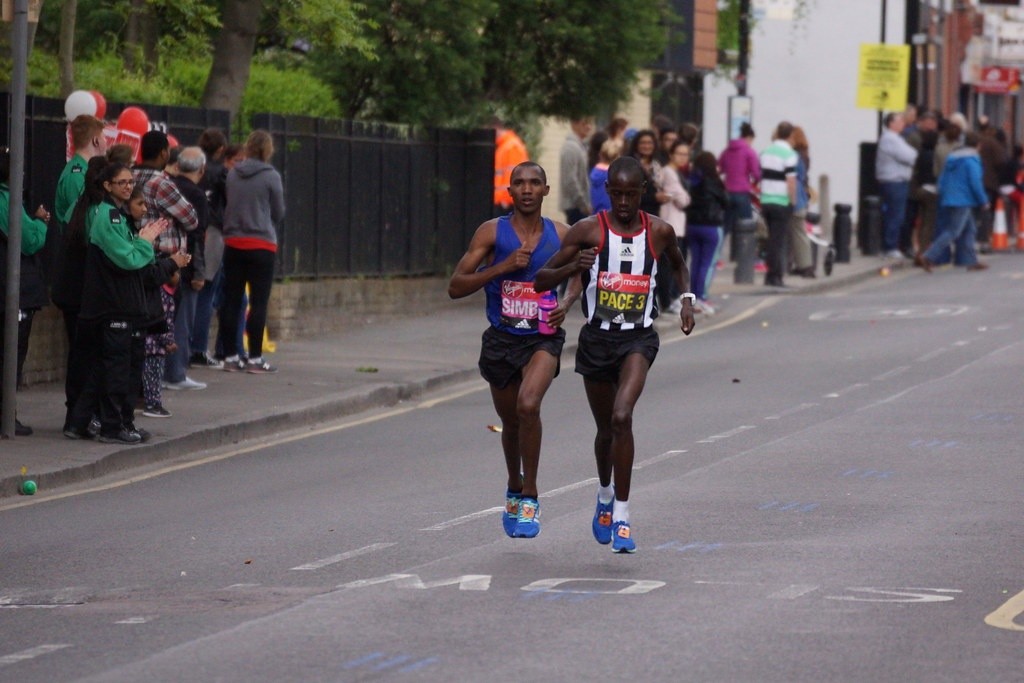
[
  {"x1": 100, "y1": 425, "x2": 151, "y2": 444},
  {"x1": 58, "y1": 417, "x2": 100, "y2": 440},
  {"x1": 141, "y1": 405, "x2": 172, "y2": 417},
  {"x1": 162, "y1": 375, "x2": 206, "y2": 391},
  {"x1": 502, "y1": 475, "x2": 541, "y2": 538},
  {"x1": 245, "y1": 359, "x2": 279, "y2": 374},
  {"x1": 222, "y1": 358, "x2": 247, "y2": 372},
  {"x1": 189, "y1": 351, "x2": 224, "y2": 369},
  {"x1": 592, "y1": 484, "x2": 636, "y2": 555}
]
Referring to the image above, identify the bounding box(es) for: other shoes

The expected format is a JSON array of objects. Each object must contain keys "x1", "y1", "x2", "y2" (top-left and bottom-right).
[
  {"x1": 764, "y1": 271, "x2": 784, "y2": 287},
  {"x1": 966, "y1": 262, "x2": 989, "y2": 271},
  {"x1": 915, "y1": 253, "x2": 931, "y2": 273}
]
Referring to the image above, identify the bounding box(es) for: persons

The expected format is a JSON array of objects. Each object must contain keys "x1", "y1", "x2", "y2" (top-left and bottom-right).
[
  {"x1": 480, "y1": 116, "x2": 529, "y2": 220},
  {"x1": 760, "y1": 122, "x2": 816, "y2": 289},
  {"x1": 718, "y1": 121, "x2": 763, "y2": 260},
  {"x1": 0, "y1": 115, "x2": 286, "y2": 443},
  {"x1": 561, "y1": 109, "x2": 730, "y2": 316},
  {"x1": 875, "y1": 105, "x2": 1024, "y2": 274},
  {"x1": 448, "y1": 162, "x2": 582, "y2": 536},
  {"x1": 533, "y1": 156, "x2": 696, "y2": 554}
]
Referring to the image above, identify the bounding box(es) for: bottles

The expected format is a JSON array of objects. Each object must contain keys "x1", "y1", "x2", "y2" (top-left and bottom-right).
[{"x1": 537, "y1": 290, "x2": 557, "y2": 334}]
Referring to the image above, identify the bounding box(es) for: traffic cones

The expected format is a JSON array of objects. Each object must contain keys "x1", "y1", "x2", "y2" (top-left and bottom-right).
[{"x1": 982, "y1": 189, "x2": 1023, "y2": 253}]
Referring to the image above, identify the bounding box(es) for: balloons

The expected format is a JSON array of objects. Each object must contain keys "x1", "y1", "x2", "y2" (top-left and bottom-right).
[
  {"x1": 64, "y1": 90, "x2": 106, "y2": 123},
  {"x1": 117, "y1": 107, "x2": 147, "y2": 165},
  {"x1": 166, "y1": 133, "x2": 178, "y2": 151}
]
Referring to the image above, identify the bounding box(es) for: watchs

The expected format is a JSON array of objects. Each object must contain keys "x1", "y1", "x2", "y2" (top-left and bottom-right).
[{"x1": 679, "y1": 293, "x2": 696, "y2": 306}]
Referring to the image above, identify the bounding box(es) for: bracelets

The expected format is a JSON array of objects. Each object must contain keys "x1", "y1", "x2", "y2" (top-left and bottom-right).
[{"x1": 664, "y1": 191, "x2": 672, "y2": 202}]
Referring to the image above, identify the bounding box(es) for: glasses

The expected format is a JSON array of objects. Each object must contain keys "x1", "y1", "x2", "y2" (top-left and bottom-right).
[{"x1": 109, "y1": 180, "x2": 137, "y2": 187}]
